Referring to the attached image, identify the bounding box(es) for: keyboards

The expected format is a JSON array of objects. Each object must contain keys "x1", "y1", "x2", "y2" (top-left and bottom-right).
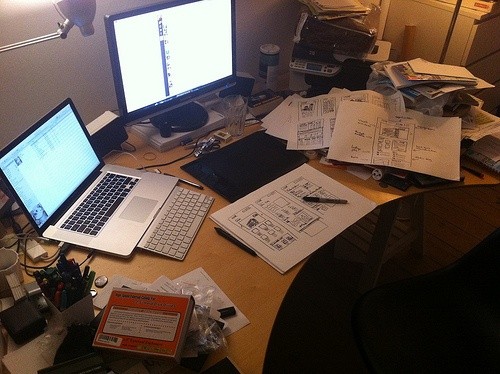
[{"x1": 135, "y1": 184, "x2": 216, "y2": 261}]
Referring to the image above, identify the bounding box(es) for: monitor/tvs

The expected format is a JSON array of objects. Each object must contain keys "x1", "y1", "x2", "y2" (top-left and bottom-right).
[{"x1": 102, "y1": 0, "x2": 239, "y2": 135}]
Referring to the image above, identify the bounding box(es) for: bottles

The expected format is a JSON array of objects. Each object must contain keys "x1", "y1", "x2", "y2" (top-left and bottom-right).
[{"x1": 258, "y1": 44, "x2": 280, "y2": 78}]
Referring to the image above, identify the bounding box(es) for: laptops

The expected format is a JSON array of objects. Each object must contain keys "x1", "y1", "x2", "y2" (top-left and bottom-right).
[{"x1": 0, "y1": 97, "x2": 180, "y2": 260}]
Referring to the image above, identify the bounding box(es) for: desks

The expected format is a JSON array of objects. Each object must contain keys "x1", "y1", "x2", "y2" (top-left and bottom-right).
[{"x1": 0, "y1": 80, "x2": 500, "y2": 374}]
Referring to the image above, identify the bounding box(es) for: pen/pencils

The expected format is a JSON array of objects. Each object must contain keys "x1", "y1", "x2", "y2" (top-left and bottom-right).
[
  {"x1": 213, "y1": 226, "x2": 258, "y2": 257},
  {"x1": 163, "y1": 172, "x2": 204, "y2": 190},
  {"x1": 302, "y1": 197, "x2": 348, "y2": 204},
  {"x1": 33, "y1": 253, "x2": 96, "y2": 311}
]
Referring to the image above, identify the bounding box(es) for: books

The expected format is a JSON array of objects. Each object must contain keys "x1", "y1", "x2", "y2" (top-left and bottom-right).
[
  {"x1": 378, "y1": 56, "x2": 477, "y2": 103},
  {"x1": 92, "y1": 290, "x2": 195, "y2": 364},
  {"x1": 364, "y1": 164, "x2": 464, "y2": 189},
  {"x1": 464, "y1": 134, "x2": 500, "y2": 175}
]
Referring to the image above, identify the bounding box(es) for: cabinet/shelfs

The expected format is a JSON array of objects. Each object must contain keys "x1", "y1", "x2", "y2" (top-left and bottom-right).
[{"x1": 384, "y1": 0, "x2": 500, "y2": 114}]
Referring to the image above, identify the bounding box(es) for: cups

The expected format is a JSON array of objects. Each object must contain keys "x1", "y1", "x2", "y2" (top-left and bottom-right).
[
  {"x1": 41, "y1": 292, "x2": 95, "y2": 330},
  {"x1": 222, "y1": 95, "x2": 248, "y2": 136},
  {"x1": 0, "y1": 247, "x2": 25, "y2": 297}
]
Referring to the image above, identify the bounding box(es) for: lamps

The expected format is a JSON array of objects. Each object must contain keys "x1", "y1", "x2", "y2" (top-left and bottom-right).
[{"x1": 0, "y1": 0, "x2": 97, "y2": 54}]
